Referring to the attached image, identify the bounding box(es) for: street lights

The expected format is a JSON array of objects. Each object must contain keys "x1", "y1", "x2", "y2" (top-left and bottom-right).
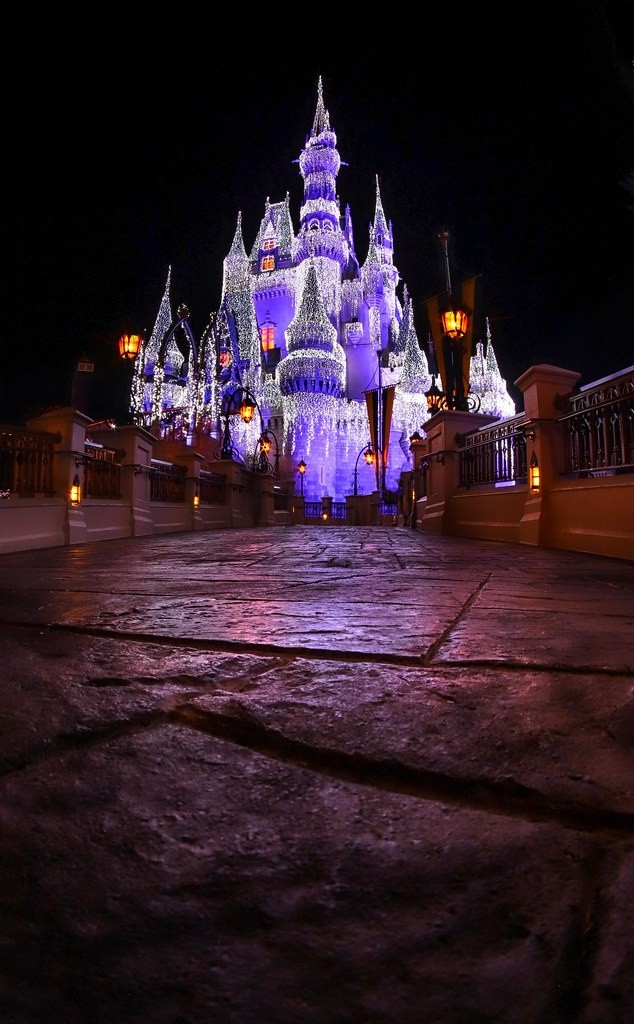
[
  {"x1": 347, "y1": 441, "x2": 376, "y2": 496},
  {"x1": 441, "y1": 298, "x2": 473, "y2": 411},
  {"x1": 214, "y1": 383, "x2": 257, "y2": 460},
  {"x1": 298, "y1": 459, "x2": 307, "y2": 496},
  {"x1": 255, "y1": 427, "x2": 281, "y2": 477},
  {"x1": 118, "y1": 327, "x2": 143, "y2": 425}
]
